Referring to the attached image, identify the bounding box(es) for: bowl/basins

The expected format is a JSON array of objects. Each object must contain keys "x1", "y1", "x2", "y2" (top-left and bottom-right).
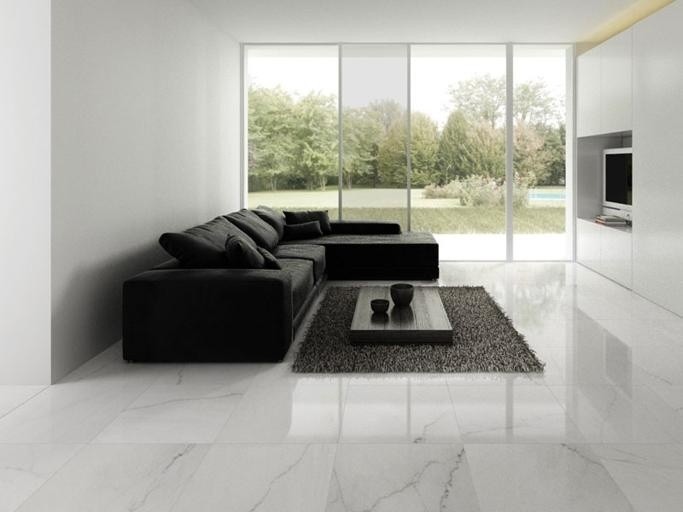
[
  {"x1": 371, "y1": 299, "x2": 389, "y2": 312},
  {"x1": 390, "y1": 284, "x2": 414, "y2": 306}
]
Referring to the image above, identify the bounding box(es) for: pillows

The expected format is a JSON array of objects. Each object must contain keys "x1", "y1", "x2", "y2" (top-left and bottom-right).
[
  {"x1": 252, "y1": 204, "x2": 285, "y2": 241},
  {"x1": 258, "y1": 247, "x2": 281, "y2": 270},
  {"x1": 159, "y1": 216, "x2": 259, "y2": 268},
  {"x1": 283, "y1": 207, "x2": 331, "y2": 234},
  {"x1": 226, "y1": 234, "x2": 264, "y2": 268},
  {"x1": 283, "y1": 220, "x2": 323, "y2": 241},
  {"x1": 223, "y1": 209, "x2": 280, "y2": 250}
]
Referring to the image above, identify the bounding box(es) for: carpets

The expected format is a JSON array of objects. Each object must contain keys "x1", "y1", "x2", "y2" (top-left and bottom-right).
[{"x1": 291, "y1": 285, "x2": 546, "y2": 374}]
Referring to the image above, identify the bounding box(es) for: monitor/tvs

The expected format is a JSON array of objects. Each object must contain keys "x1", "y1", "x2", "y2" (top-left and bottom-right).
[{"x1": 602, "y1": 147, "x2": 632, "y2": 221}]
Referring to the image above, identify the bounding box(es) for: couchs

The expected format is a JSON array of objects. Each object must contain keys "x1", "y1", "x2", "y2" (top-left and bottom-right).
[{"x1": 122, "y1": 220, "x2": 439, "y2": 363}]
